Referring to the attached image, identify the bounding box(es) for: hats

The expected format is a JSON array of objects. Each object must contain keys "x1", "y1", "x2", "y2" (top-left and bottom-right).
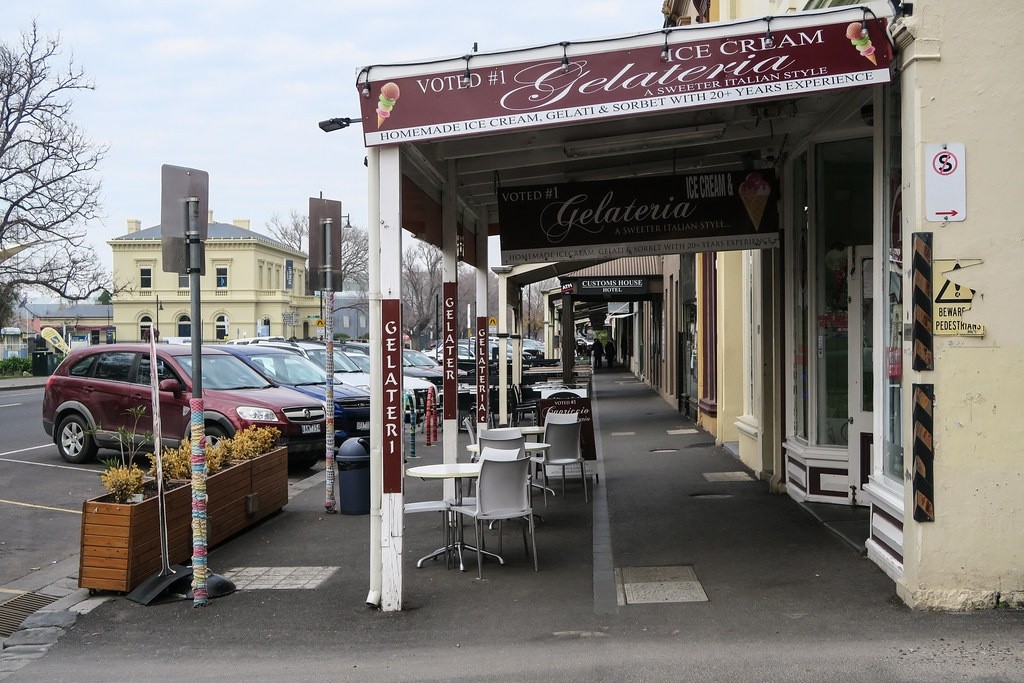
[{"x1": 609, "y1": 340, "x2": 612, "y2": 343}]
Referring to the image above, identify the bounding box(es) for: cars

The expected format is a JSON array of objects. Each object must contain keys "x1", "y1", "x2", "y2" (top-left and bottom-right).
[
  {"x1": 202, "y1": 345, "x2": 369, "y2": 454},
  {"x1": 224, "y1": 337, "x2": 546, "y2": 422}
]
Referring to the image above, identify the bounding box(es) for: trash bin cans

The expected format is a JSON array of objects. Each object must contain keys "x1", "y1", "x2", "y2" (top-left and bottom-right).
[
  {"x1": 31, "y1": 351, "x2": 52, "y2": 377},
  {"x1": 335, "y1": 437, "x2": 370, "y2": 516},
  {"x1": 52, "y1": 353, "x2": 58, "y2": 373}
]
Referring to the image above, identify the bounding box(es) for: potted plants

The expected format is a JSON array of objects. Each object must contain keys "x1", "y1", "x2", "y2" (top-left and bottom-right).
[{"x1": 79, "y1": 424, "x2": 289, "y2": 593}]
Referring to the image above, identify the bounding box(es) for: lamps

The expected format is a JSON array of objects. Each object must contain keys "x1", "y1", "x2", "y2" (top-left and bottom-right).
[
  {"x1": 563, "y1": 123, "x2": 727, "y2": 157},
  {"x1": 319, "y1": 118, "x2": 362, "y2": 132}
]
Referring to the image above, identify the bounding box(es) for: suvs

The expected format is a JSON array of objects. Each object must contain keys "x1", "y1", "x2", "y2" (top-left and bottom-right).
[{"x1": 42, "y1": 341, "x2": 328, "y2": 473}]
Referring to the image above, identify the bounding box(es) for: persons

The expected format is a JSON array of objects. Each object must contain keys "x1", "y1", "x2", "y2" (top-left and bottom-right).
[
  {"x1": 605, "y1": 340, "x2": 616, "y2": 367},
  {"x1": 591, "y1": 338, "x2": 605, "y2": 368}
]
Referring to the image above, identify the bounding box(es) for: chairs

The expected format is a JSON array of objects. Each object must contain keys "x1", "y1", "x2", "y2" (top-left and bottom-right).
[
  {"x1": 414, "y1": 385, "x2": 476, "y2": 434},
  {"x1": 488, "y1": 384, "x2": 539, "y2": 429},
  {"x1": 403, "y1": 413, "x2": 588, "y2": 577}
]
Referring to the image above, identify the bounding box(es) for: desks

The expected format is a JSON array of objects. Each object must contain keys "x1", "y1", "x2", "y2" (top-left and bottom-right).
[
  {"x1": 439, "y1": 391, "x2": 477, "y2": 394},
  {"x1": 441, "y1": 388, "x2": 477, "y2": 391},
  {"x1": 459, "y1": 385, "x2": 495, "y2": 389},
  {"x1": 530, "y1": 382, "x2": 588, "y2": 425},
  {"x1": 466, "y1": 442, "x2": 551, "y2": 529},
  {"x1": 406, "y1": 463, "x2": 506, "y2": 573},
  {"x1": 490, "y1": 426, "x2": 556, "y2": 497}
]
